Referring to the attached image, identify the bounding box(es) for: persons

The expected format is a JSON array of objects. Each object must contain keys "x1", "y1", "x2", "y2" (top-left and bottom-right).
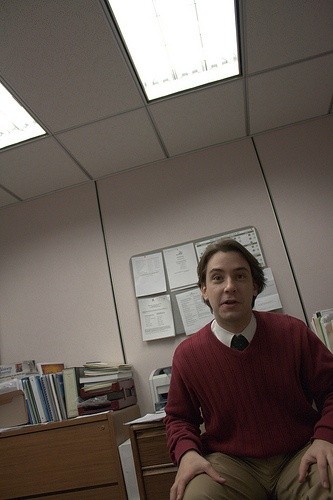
[{"x1": 163, "y1": 239, "x2": 333, "y2": 500}]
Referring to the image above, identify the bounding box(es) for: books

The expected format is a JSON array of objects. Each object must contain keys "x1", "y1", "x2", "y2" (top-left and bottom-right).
[
  {"x1": 0, "y1": 358, "x2": 137, "y2": 427},
  {"x1": 123, "y1": 413, "x2": 168, "y2": 426}
]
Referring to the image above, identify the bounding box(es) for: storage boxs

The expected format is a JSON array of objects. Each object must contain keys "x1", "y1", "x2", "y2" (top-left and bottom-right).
[{"x1": 0, "y1": 389, "x2": 29, "y2": 428}]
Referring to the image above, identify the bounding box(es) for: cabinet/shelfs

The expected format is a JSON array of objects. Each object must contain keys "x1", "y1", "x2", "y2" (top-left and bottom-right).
[
  {"x1": 0, "y1": 404, "x2": 143, "y2": 500},
  {"x1": 128, "y1": 421, "x2": 178, "y2": 500}
]
